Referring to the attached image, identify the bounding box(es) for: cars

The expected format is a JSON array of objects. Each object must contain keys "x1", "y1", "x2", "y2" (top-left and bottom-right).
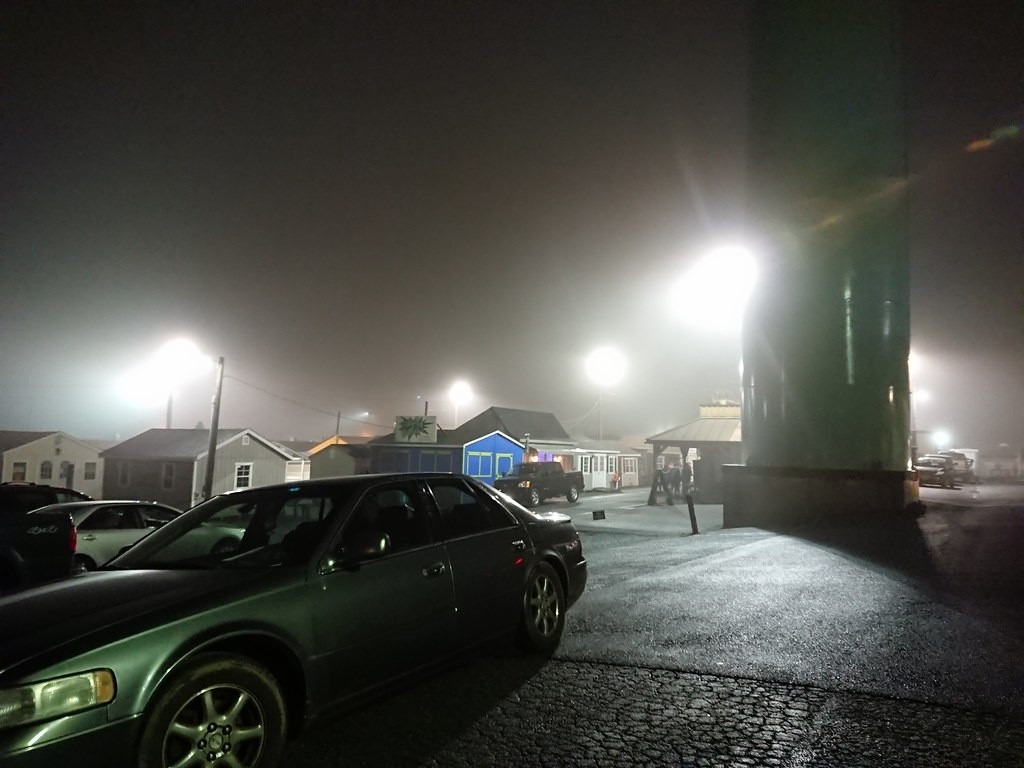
[
  {"x1": 11, "y1": 500, "x2": 248, "y2": 574},
  {"x1": 925, "y1": 454, "x2": 958, "y2": 478},
  {"x1": 1, "y1": 471, "x2": 590, "y2": 768},
  {"x1": 914, "y1": 456, "x2": 952, "y2": 488},
  {"x1": 1, "y1": 482, "x2": 97, "y2": 523},
  {"x1": 938, "y1": 450, "x2": 974, "y2": 482}
]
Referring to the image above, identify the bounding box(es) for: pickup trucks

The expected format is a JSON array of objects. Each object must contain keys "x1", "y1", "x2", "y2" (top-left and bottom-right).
[{"x1": 494, "y1": 461, "x2": 586, "y2": 508}]
